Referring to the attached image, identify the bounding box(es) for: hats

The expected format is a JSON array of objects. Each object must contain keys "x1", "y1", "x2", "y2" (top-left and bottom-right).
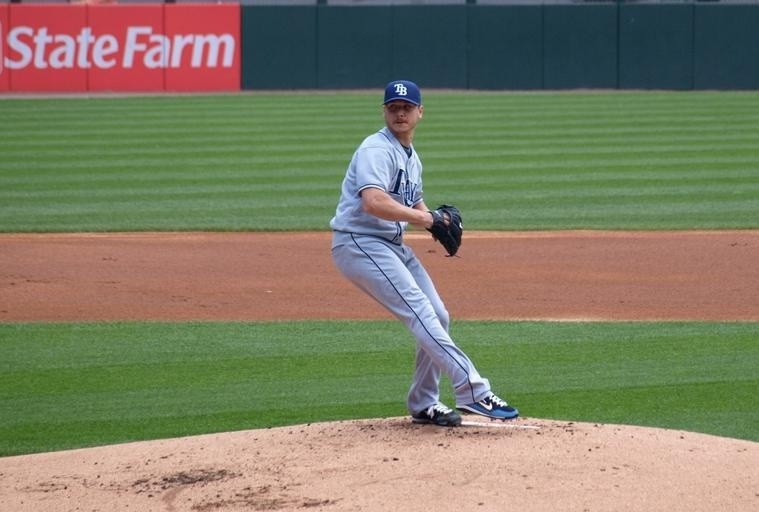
[{"x1": 382, "y1": 80, "x2": 421, "y2": 106}]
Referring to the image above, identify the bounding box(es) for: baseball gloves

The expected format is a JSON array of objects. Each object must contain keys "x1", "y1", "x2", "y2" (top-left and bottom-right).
[{"x1": 426, "y1": 205, "x2": 462, "y2": 256}]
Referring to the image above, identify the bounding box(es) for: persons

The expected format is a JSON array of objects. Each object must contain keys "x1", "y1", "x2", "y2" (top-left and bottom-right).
[{"x1": 328, "y1": 78, "x2": 522, "y2": 428}]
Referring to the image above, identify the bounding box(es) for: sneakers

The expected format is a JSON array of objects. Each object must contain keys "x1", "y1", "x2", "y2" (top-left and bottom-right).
[
  {"x1": 455, "y1": 394, "x2": 519, "y2": 419},
  {"x1": 412, "y1": 401, "x2": 460, "y2": 426}
]
